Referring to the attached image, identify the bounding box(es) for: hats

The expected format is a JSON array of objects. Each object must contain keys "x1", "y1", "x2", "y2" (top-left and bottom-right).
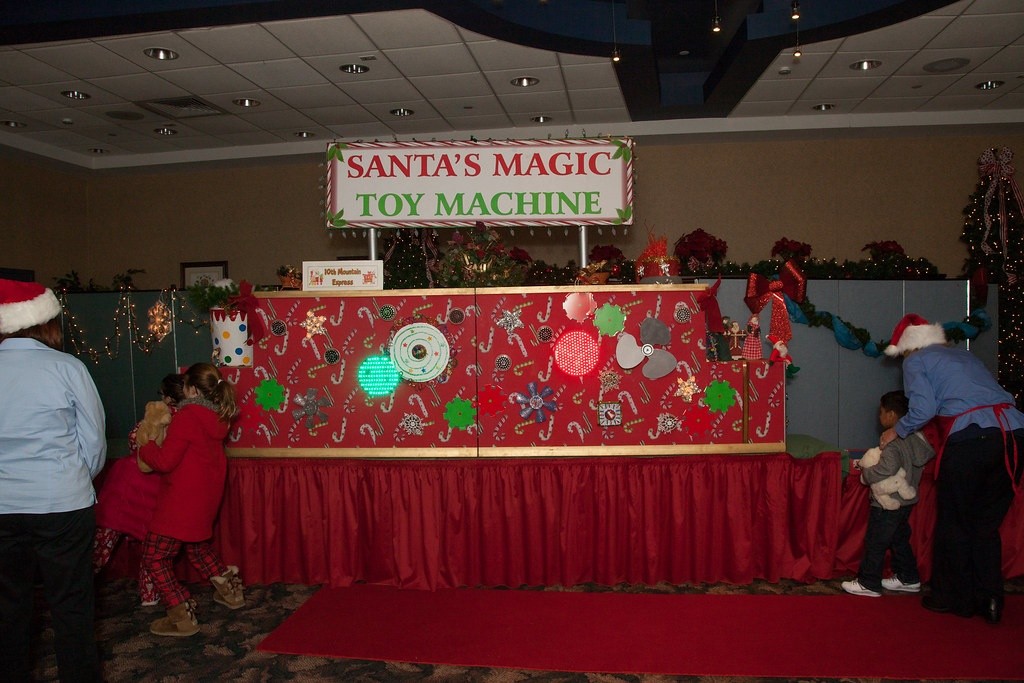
[
  {"x1": 884, "y1": 312, "x2": 946, "y2": 356},
  {"x1": 1, "y1": 279, "x2": 62, "y2": 335}
]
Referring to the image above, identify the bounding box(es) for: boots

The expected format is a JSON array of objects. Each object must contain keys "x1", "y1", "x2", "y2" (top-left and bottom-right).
[
  {"x1": 149, "y1": 602, "x2": 201, "y2": 636},
  {"x1": 211, "y1": 564, "x2": 247, "y2": 608}
]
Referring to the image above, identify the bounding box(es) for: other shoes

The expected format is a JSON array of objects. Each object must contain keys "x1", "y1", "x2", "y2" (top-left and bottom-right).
[{"x1": 921, "y1": 589, "x2": 1002, "y2": 624}]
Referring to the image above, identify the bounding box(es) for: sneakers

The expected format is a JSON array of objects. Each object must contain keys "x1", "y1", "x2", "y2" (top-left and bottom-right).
[
  {"x1": 882, "y1": 575, "x2": 921, "y2": 592},
  {"x1": 842, "y1": 579, "x2": 882, "y2": 597}
]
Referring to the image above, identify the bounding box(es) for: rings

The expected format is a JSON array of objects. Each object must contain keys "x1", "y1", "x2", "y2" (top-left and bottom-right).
[{"x1": 881, "y1": 442, "x2": 883, "y2": 445}]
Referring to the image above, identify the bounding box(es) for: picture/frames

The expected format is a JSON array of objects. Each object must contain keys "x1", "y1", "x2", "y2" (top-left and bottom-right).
[{"x1": 179, "y1": 261, "x2": 229, "y2": 290}]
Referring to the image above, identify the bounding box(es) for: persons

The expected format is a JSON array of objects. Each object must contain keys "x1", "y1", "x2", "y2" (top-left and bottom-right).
[
  {"x1": 879, "y1": 312, "x2": 1024, "y2": 625},
  {"x1": 842, "y1": 392, "x2": 921, "y2": 597},
  {"x1": 88, "y1": 362, "x2": 246, "y2": 638},
  {"x1": 0, "y1": 278, "x2": 109, "y2": 683}
]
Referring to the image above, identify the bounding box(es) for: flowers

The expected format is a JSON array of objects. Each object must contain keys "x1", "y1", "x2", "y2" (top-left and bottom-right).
[
  {"x1": 675, "y1": 229, "x2": 729, "y2": 263},
  {"x1": 770, "y1": 236, "x2": 811, "y2": 261},
  {"x1": 861, "y1": 240, "x2": 906, "y2": 261},
  {"x1": 432, "y1": 226, "x2": 533, "y2": 285}
]
